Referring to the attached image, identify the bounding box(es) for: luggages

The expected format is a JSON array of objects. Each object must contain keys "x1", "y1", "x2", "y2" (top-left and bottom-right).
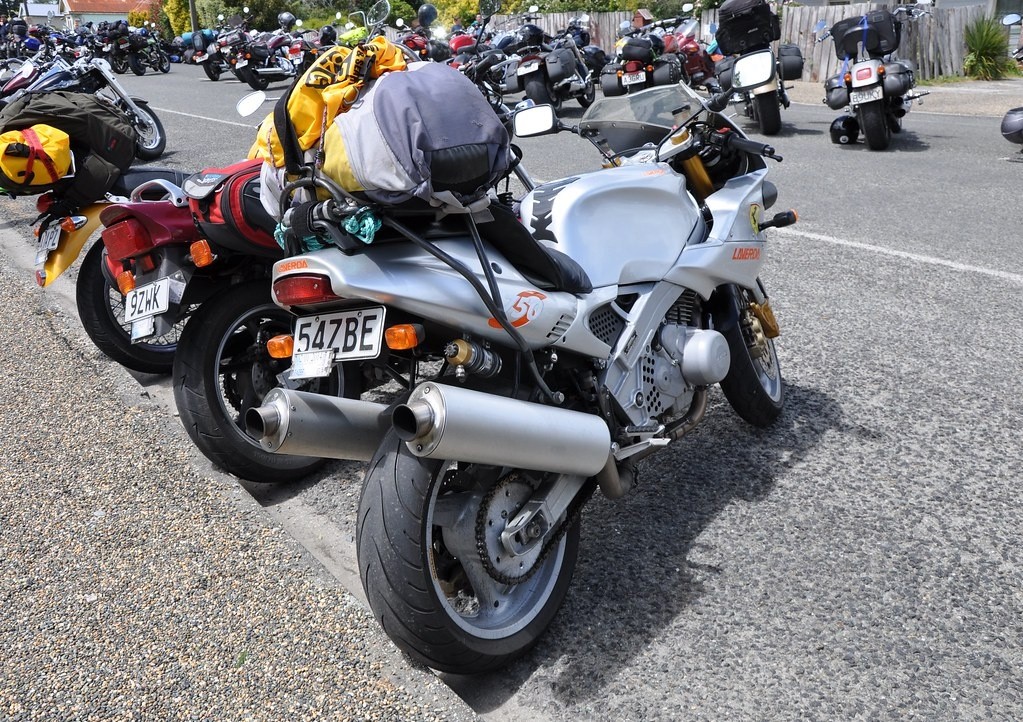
[
  {"x1": 831, "y1": 11, "x2": 900, "y2": 60},
  {"x1": 714, "y1": 0, "x2": 781, "y2": 57}
]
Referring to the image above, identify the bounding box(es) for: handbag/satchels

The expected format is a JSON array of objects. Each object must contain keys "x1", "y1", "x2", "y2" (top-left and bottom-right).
[
  {"x1": 0, "y1": 86, "x2": 137, "y2": 216},
  {"x1": 180, "y1": 42, "x2": 512, "y2": 256},
  {"x1": 97, "y1": 20, "x2": 128, "y2": 37}
]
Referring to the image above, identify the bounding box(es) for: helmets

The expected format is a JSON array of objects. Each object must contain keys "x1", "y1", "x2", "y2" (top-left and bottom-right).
[
  {"x1": 517, "y1": 24, "x2": 544, "y2": 49},
  {"x1": 418, "y1": 4, "x2": 438, "y2": 27},
  {"x1": 569, "y1": 16, "x2": 582, "y2": 28},
  {"x1": 278, "y1": 12, "x2": 296, "y2": 30},
  {"x1": 318, "y1": 26, "x2": 338, "y2": 45}
]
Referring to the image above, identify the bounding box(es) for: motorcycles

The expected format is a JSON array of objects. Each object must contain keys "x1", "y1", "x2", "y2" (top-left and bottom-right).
[
  {"x1": 999, "y1": 11, "x2": 1023, "y2": 144},
  {"x1": 812, "y1": 0, "x2": 936, "y2": 151},
  {"x1": 0, "y1": 0, "x2": 806, "y2": 674}
]
